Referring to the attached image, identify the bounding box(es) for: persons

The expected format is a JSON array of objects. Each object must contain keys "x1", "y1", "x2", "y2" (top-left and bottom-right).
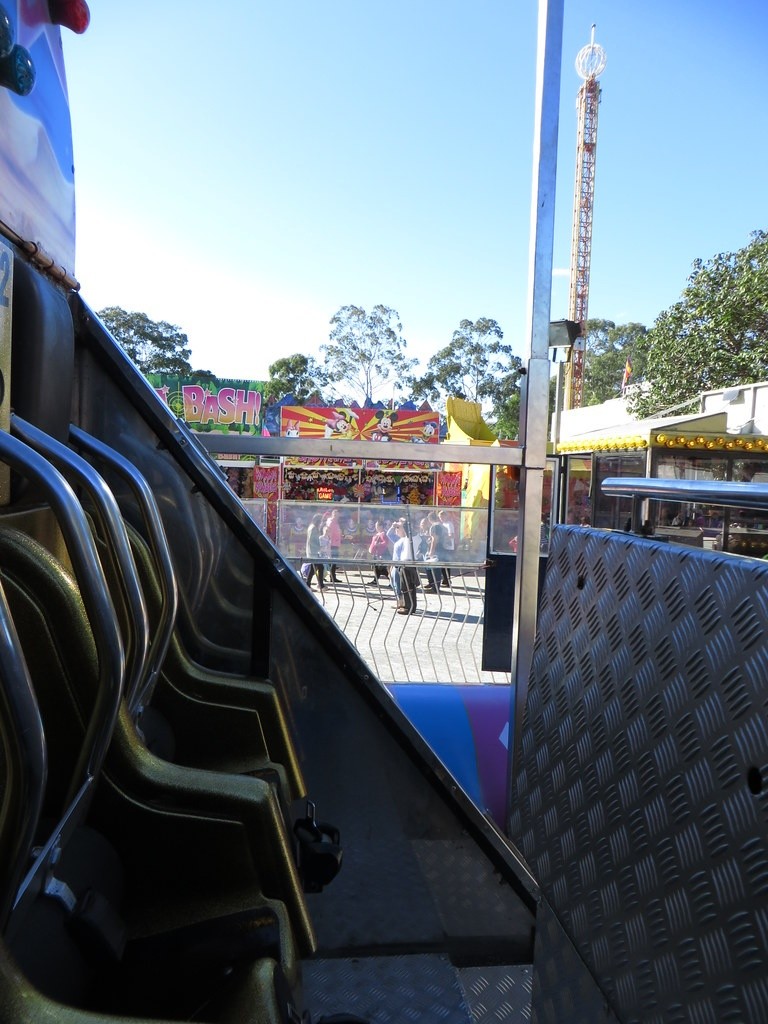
[{"x1": 299, "y1": 508, "x2": 454, "y2": 615}]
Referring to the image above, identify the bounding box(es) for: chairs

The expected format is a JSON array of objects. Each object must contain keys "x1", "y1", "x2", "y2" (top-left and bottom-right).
[{"x1": 0, "y1": 412, "x2": 340, "y2": 1024}]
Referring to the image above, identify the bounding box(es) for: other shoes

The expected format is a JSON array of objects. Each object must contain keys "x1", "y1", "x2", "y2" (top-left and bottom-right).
[
  {"x1": 309, "y1": 587, "x2": 318, "y2": 592},
  {"x1": 397, "y1": 609, "x2": 412, "y2": 615},
  {"x1": 316, "y1": 584, "x2": 328, "y2": 591}
]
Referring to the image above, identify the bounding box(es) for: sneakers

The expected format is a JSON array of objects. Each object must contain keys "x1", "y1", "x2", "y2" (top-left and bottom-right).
[{"x1": 367, "y1": 580, "x2": 379, "y2": 586}]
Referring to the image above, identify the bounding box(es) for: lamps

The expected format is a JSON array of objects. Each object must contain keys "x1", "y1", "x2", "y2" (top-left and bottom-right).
[{"x1": 547, "y1": 319, "x2": 583, "y2": 349}]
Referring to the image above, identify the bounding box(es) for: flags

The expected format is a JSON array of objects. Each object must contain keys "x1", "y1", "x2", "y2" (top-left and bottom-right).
[{"x1": 622, "y1": 357, "x2": 633, "y2": 388}]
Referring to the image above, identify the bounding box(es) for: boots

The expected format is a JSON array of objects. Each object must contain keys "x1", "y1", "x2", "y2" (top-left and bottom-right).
[{"x1": 330, "y1": 574, "x2": 343, "y2": 583}]
